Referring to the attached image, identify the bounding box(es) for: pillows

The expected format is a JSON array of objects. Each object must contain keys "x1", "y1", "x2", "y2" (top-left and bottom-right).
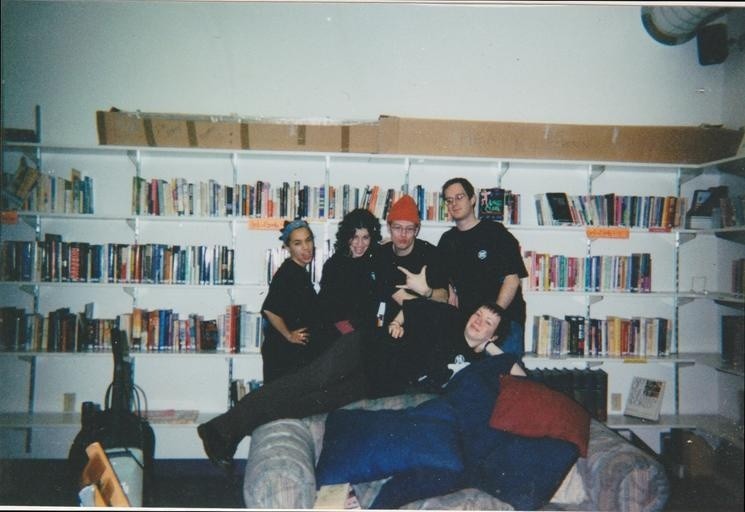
[{"x1": 316, "y1": 351, "x2": 593, "y2": 512}]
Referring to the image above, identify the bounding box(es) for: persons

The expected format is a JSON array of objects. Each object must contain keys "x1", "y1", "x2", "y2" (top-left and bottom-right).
[
  {"x1": 261, "y1": 178, "x2": 528, "y2": 380},
  {"x1": 198, "y1": 298, "x2": 530, "y2": 478}
]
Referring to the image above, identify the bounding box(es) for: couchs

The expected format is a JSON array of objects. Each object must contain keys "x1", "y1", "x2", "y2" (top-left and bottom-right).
[{"x1": 242, "y1": 391, "x2": 673, "y2": 511}]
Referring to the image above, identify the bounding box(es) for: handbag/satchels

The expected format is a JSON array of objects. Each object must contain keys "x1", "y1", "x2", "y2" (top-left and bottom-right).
[{"x1": 69, "y1": 383, "x2": 154, "y2": 466}]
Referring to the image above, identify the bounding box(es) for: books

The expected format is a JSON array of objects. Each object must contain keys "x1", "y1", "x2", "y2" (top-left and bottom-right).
[
  {"x1": 292, "y1": 181, "x2": 744, "y2": 226},
  {"x1": 517, "y1": 247, "x2": 651, "y2": 293},
  {"x1": 0, "y1": 239, "x2": 327, "y2": 284},
  {"x1": 0, "y1": 169, "x2": 93, "y2": 214},
  {"x1": 530, "y1": 314, "x2": 672, "y2": 357},
  {"x1": 624, "y1": 375, "x2": 666, "y2": 421},
  {"x1": 720, "y1": 258, "x2": 745, "y2": 365},
  {"x1": 230, "y1": 377, "x2": 264, "y2": 407},
  {"x1": 1, "y1": 304, "x2": 292, "y2": 352},
  {"x1": 132, "y1": 176, "x2": 300, "y2": 217}
]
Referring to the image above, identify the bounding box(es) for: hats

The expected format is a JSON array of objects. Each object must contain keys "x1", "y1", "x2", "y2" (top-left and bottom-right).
[{"x1": 388, "y1": 195, "x2": 419, "y2": 225}]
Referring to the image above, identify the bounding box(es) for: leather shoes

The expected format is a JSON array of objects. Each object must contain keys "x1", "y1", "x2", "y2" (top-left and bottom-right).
[{"x1": 198, "y1": 424, "x2": 235, "y2": 470}]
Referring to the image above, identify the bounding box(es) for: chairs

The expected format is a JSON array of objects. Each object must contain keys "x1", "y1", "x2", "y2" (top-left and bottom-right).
[{"x1": 78, "y1": 440, "x2": 135, "y2": 508}]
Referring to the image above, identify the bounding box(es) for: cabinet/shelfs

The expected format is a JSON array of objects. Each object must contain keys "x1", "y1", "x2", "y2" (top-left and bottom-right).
[{"x1": 0, "y1": 141, "x2": 745, "y2": 455}]
